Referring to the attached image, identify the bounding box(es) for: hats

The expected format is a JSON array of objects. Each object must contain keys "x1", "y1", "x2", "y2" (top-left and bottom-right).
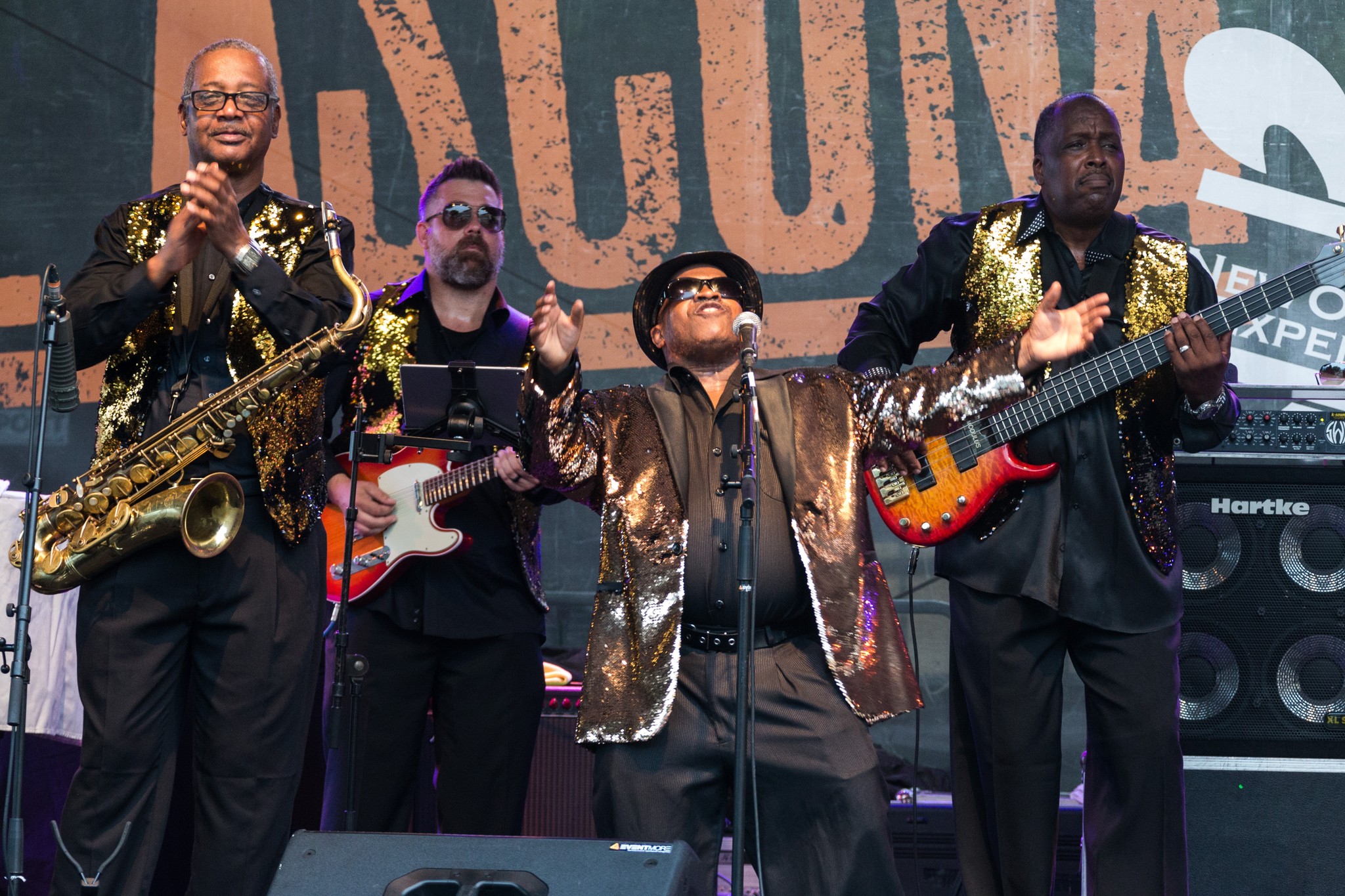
[{"x1": 632, "y1": 251, "x2": 764, "y2": 371}]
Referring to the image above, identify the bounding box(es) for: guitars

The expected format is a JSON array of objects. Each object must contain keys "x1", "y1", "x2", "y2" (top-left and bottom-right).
[
  {"x1": 865, "y1": 243, "x2": 1344, "y2": 543},
  {"x1": 307, "y1": 440, "x2": 532, "y2": 603}
]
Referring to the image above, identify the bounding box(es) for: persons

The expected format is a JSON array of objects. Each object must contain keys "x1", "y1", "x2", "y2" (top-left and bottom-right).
[
  {"x1": 515, "y1": 248, "x2": 1109, "y2": 896},
  {"x1": 61, "y1": 41, "x2": 357, "y2": 895},
  {"x1": 322, "y1": 158, "x2": 606, "y2": 837},
  {"x1": 837, "y1": 85, "x2": 1237, "y2": 896}
]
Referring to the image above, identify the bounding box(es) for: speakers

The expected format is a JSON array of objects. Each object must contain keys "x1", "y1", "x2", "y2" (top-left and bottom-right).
[
  {"x1": 265, "y1": 830, "x2": 714, "y2": 896},
  {"x1": 864, "y1": 485, "x2": 1090, "y2": 799},
  {"x1": 536, "y1": 498, "x2": 603, "y2": 653},
  {"x1": 1182, "y1": 754, "x2": 1345, "y2": 895},
  {"x1": 1174, "y1": 453, "x2": 1345, "y2": 759}
]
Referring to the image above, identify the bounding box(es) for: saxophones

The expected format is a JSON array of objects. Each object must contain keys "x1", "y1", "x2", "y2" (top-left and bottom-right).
[{"x1": 8, "y1": 203, "x2": 370, "y2": 596}]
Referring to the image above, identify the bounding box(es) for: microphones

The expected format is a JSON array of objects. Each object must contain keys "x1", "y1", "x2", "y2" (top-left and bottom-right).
[
  {"x1": 732, "y1": 311, "x2": 761, "y2": 378},
  {"x1": 44, "y1": 270, "x2": 79, "y2": 413}
]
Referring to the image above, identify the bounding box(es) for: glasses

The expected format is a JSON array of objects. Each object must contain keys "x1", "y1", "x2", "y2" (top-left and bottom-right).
[
  {"x1": 656, "y1": 277, "x2": 746, "y2": 324},
  {"x1": 425, "y1": 202, "x2": 507, "y2": 233},
  {"x1": 181, "y1": 89, "x2": 280, "y2": 113}
]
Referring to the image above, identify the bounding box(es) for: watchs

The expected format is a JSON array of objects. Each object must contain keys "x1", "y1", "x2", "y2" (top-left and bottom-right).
[
  {"x1": 226, "y1": 238, "x2": 263, "y2": 276},
  {"x1": 1179, "y1": 386, "x2": 1227, "y2": 421}
]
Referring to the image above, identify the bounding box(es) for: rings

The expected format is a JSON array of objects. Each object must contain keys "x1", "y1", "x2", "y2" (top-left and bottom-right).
[
  {"x1": 511, "y1": 475, "x2": 521, "y2": 482},
  {"x1": 1180, "y1": 345, "x2": 1191, "y2": 353}
]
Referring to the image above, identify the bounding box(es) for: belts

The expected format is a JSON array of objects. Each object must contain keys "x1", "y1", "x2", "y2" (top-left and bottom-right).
[{"x1": 680, "y1": 620, "x2": 808, "y2": 653}]
